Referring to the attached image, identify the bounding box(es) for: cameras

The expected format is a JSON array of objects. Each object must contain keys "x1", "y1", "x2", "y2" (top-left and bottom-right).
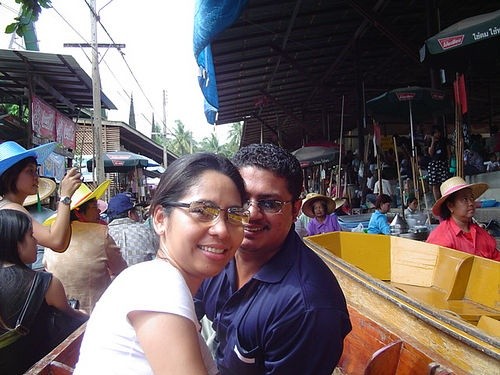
[{"x1": 66, "y1": 168, "x2": 84, "y2": 182}]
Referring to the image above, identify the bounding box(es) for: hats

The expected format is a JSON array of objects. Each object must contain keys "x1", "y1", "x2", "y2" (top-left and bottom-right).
[
  {"x1": 106, "y1": 194, "x2": 133, "y2": 216},
  {"x1": 23, "y1": 177, "x2": 55, "y2": 206},
  {"x1": 302, "y1": 195, "x2": 336, "y2": 218},
  {"x1": 433, "y1": 176, "x2": 488, "y2": 218},
  {"x1": 42, "y1": 179, "x2": 110, "y2": 226},
  {"x1": 0, "y1": 140, "x2": 56, "y2": 175}
]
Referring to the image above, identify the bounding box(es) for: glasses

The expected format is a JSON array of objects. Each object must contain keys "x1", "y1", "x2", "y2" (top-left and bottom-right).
[
  {"x1": 161, "y1": 199, "x2": 251, "y2": 228},
  {"x1": 243, "y1": 199, "x2": 295, "y2": 214}
]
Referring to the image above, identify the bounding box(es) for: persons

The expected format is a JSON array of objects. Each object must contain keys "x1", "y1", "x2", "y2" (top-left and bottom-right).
[
  {"x1": 108, "y1": 194, "x2": 156, "y2": 261},
  {"x1": 423, "y1": 125, "x2": 450, "y2": 200},
  {"x1": 46, "y1": 178, "x2": 128, "y2": 313},
  {"x1": 0, "y1": 141, "x2": 82, "y2": 250},
  {"x1": 303, "y1": 195, "x2": 344, "y2": 234},
  {"x1": 463, "y1": 148, "x2": 484, "y2": 174},
  {"x1": 484, "y1": 153, "x2": 500, "y2": 172},
  {"x1": 311, "y1": 129, "x2": 421, "y2": 207},
  {"x1": 424, "y1": 175, "x2": 500, "y2": 261},
  {"x1": 25, "y1": 175, "x2": 58, "y2": 269},
  {"x1": 77, "y1": 152, "x2": 246, "y2": 374},
  {"x1": 368, "y1": 193, "x2": 392, "y2": 235},
  {"x1": 0, "y1": 208, "x2": 89, "y2": 374},
  {"x1": 402, "y1": 197, "x2": 419, "y2": 217},
  {"x1": 193, "y1": 143, "x2": 353, "y2": 374},
  {"x1": 144, "y1": 216, "x2": 159, "y2": 252}
]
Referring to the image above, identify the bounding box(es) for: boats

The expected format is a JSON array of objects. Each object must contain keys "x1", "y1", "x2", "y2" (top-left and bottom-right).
[
  {"x1": 23, "y1": 321, "x2": 92, "y2": 375},
  {"x1": 304, "y1": 232, "x2": 500, "y2": 375}
]
